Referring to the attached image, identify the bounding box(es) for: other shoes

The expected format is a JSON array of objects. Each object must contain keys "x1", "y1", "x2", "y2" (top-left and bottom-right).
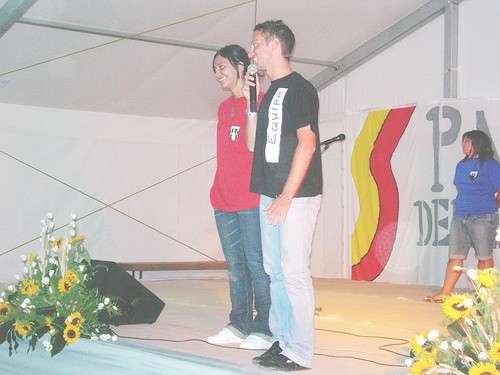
[
  {"x1": 252, "y1": 341, "x2": 282, "y2": 363},
  {"x1": 259, "y1": 353, "x2": 308, "y2": 371}
]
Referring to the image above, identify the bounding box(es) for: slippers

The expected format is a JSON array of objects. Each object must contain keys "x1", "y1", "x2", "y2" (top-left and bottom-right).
[{"x1": 426, "y1": 295, "x2": 444, "y2": 302}]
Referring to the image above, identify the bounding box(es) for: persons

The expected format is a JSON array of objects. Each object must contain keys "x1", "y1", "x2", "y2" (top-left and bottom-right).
[
  {"x1": 423, "y1": 130, "x2": 500, "y2": 303},
  {"x1": 241, "y1": 18, "x2": 323, "y2": 373},
  {"x1": 205, "y1": 45, "x2": 274, "y2": 348}
]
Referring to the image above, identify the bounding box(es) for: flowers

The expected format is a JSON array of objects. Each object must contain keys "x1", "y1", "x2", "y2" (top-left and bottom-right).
[
  {"x1": 405, "y1": 266, "x2": 500, "y2": 375},
  {"x1": 0, "y1": 212, "x2": 124, "y2": 358}
]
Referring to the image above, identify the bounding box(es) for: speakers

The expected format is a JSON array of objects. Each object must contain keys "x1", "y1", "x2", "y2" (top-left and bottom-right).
[{"x1": 82, "y1": 260, "x2": 165, "y2": 326}]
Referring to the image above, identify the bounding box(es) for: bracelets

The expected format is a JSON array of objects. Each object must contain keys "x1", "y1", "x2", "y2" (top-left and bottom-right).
[
  {"x1": 246, "y1": 112, "x2": 257, "y2": 115},
  {"x1": 276, "y1": 194, "x2": 292, "y2": 203}
]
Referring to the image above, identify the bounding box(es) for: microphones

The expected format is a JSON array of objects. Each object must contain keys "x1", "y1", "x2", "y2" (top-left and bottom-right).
[
  {"x1": 247, "y1": 64, "x2": 257, "y2": 116},
  {"x1": 320, "y1": 134, "x2": 345, "y2": 145}
]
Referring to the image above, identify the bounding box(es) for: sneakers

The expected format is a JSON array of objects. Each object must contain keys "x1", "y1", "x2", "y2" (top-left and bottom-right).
[
  {"x1": 207, "y1": 328, "x2": 242, "y2": 344},
  {"x1": 239, "y1": 335, "x2": 270, "y2": 348}
]
